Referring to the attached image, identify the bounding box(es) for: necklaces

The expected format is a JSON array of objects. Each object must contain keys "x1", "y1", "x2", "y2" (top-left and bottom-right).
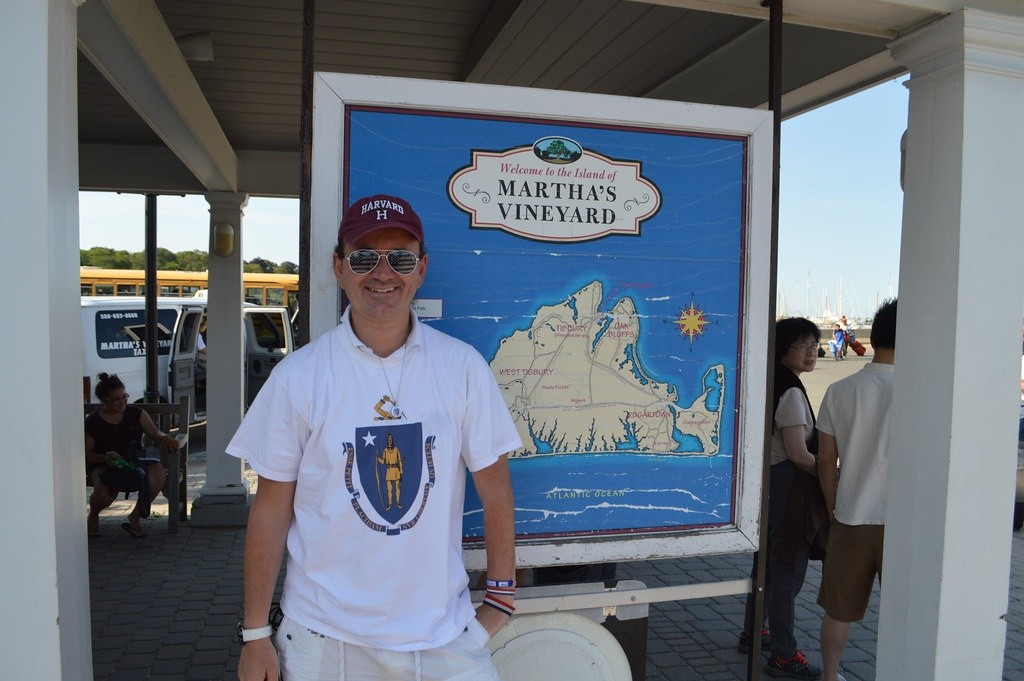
[{"x1": 364, "y1": 339, "x2": 409, "y2": 419}]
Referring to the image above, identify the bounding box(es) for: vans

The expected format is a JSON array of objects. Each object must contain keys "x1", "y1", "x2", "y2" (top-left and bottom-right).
[{"x1": 80, "y1": 289, "x2": 295, "y2": 439}]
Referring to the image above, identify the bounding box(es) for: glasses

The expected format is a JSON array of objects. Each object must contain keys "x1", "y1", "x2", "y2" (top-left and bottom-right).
[
  {"x1": 104, "y1": 393, "x2": 129, "y2": 401},
  {"x1": 344, "y1": 249, "x2": 422, "y2": 275},
  {"x1": 789, "y1": 342, "x2": 821, "y2": 353}
]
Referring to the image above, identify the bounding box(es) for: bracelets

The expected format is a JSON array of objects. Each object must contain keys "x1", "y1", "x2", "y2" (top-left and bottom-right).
[
  {"x1": 486, "y1": 580, "x2": 516, "y2": 596},
  {"x1": 483, "y1": 593, "x2": 516, "y2": 618}
]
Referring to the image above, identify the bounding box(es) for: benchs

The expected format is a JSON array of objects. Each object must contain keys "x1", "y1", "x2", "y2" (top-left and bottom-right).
[{"x1": 84, "y1": 395, "x2": 191, "y2": 534}]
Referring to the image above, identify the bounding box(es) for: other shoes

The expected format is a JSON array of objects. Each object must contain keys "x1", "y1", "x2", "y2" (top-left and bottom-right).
[
  {"x1": 817, "y1": 673, "x2": 846, "y2": 681},
  {"x1": 835, "y1": 357, "x2": 845, "y2": 361}
]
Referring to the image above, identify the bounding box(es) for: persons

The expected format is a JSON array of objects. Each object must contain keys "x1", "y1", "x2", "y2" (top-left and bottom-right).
[
  {"x1": 225, "y1": 194, "x2": 524, "y2": 681},
  {"x1": 815, "y1": 298, "x2": 898, "y2": 681},
  {"x1": 196, "y1": 315, "x2": 207, "y2": 369},
  {"x1": 737, "y1": 317, "x2": 830, "y2": 681},
  {"x1": 84, "y1": 373, "x2": 180, "y2": 539},
  {"x1": 832, "y1": 317, "x2": 855, "y2": 362}
]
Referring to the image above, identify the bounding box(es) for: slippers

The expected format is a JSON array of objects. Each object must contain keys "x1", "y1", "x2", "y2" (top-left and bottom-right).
[
  {"x1": 88, "y1": 516, "x2": 99, "y2": 536},
  {"x1": 121, "y1": 523, "x2": 147, "y2": 538}
]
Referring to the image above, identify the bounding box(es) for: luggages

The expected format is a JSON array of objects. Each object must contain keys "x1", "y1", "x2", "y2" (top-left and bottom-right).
[
  {"x1": 818, "y1": 348, "x2": 826, "y2": 357},
  {"x1": 849, "y1": 340, "x2": 866, "y2": 356}
]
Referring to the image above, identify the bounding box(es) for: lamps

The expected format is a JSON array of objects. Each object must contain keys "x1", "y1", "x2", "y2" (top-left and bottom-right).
[
  {"x1": 213, "y1": 223, "x2": 235, "y2": 257},
  {"x1": 176, "y1": 31, "x2": 215, "y2": 62}
]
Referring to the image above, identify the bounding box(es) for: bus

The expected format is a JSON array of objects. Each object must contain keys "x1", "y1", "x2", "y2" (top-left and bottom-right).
[{"x1": 79, "y1": 265, "x2": 300, "y2": 351}]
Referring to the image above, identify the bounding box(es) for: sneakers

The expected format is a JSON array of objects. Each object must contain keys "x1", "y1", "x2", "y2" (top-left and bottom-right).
[
  {"x1": 763, "y1": 648, "x2": 823, "y2": 679},
  {"x1": 738, "y1": 627, "x2": 771, "y2": 652}
]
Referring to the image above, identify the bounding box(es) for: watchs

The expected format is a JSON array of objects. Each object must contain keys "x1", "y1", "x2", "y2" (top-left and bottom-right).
[{"x1": 235, "y1": 622, "x2": 272, "y2": 643}]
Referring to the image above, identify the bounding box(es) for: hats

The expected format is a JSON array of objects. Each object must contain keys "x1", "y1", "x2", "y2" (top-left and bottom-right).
[{"x1": 338, "y1": 195, "x2": 424, "y2": 248}]
